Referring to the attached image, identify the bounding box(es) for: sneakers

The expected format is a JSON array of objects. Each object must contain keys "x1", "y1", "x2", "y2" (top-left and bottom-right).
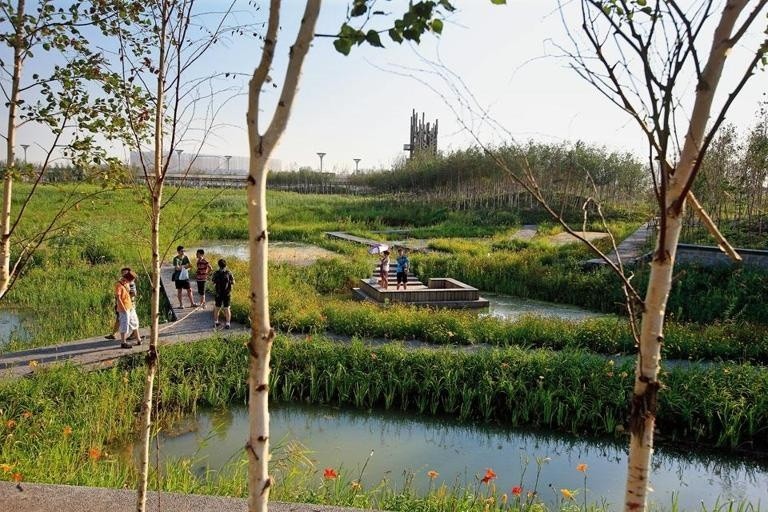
[
  {"x1": 176, "y1": 303, "x2": 231, "y2": 330},
  {"x1": 104, "y1": 332, "x2": 148, "y2": 349}
]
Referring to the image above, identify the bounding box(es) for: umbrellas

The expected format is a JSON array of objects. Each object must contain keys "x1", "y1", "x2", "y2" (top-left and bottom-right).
[{"x1": 367, "y1": 243, "x2": 388, "y2": 256}]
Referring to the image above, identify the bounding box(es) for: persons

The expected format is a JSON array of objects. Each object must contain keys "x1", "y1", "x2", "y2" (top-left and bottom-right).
[
  {"x1": 212, "y1": 258, "x2": 235, "y2": 329},
  {"x1": 194, "y1": 249, "x2": 214, "y2": 309},
  {"x1": 378, "y1": 249, "x2": 390, "y2": 289},
  {"x1": 115, "y1": 270, "x2": 147, "y2": 348},
  {"x1": 104, "y1": 267, "x2": 137, "y2": 341},
  {"x1": 396, "y1": 248, "x2": 410, "y2": 291},
  {"x1": 172, "y1": 246, "x2": 200, "y2": 309}
]
